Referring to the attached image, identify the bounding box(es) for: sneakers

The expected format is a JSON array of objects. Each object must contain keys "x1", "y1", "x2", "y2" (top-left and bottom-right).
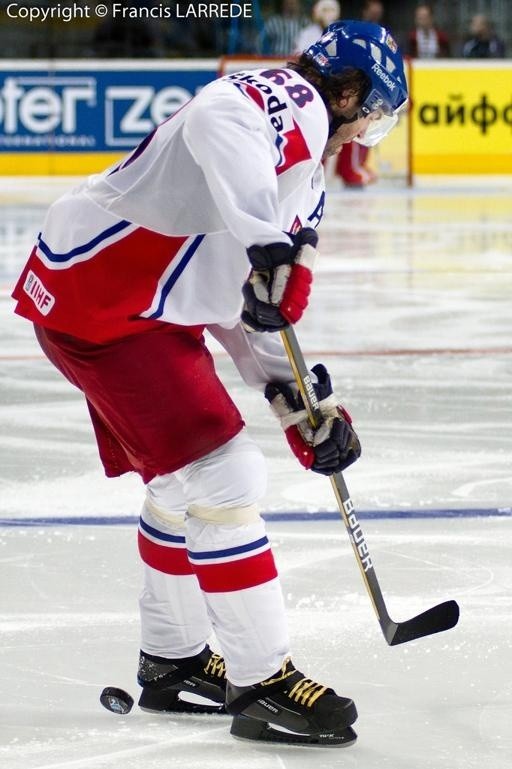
[
  {"x1": 136, "y1": 643, "x2": 228, "y2": 706},
  {"x1": 224, "y1": 655, "x2": 359, "y2": 735}
]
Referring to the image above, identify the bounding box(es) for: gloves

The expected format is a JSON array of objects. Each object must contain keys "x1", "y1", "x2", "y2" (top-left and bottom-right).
[
  {"x1": 239, "y1": 227, "x2": 320, "y2": 335},
  {"x1": 264, "y1": 362, "x2": 363, "y2": 477}
]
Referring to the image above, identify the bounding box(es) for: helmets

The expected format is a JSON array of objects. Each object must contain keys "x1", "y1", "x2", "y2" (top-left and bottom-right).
[{"x1": 305, "y1": 19, "x2": 410, "y2": 148}]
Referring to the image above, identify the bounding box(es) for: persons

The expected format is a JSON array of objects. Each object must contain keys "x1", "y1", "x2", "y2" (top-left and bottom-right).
[{"x1": 9, "y1": 19, "x2": 411, "y2": 736}]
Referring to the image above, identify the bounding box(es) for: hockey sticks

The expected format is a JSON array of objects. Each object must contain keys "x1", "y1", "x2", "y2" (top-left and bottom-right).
[{"x1": 281, "y1": 328, "x2": 460, "y2": 646}]
